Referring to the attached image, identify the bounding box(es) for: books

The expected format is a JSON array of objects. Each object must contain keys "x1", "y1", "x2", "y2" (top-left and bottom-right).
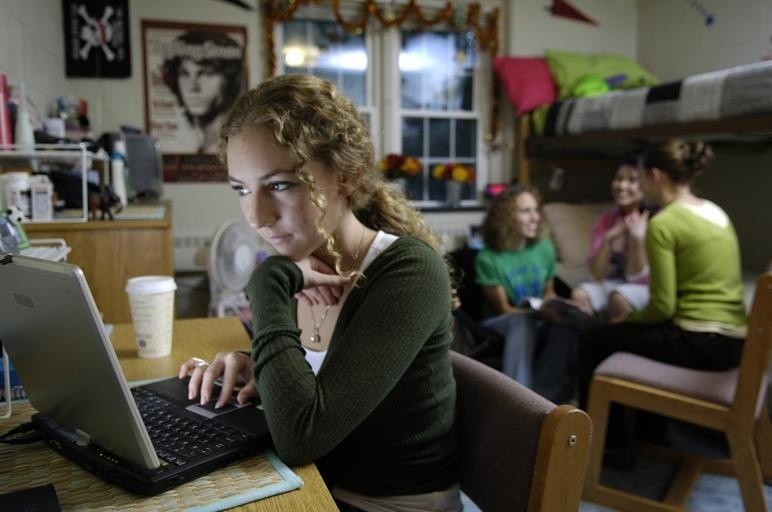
[{"x1": 514, "y1": 297, "x2": 599, "y2": 325}]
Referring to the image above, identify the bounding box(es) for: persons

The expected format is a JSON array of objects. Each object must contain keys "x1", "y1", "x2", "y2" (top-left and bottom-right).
[
  {"x1": 477, "y1": 184, "x2": 580, "y2": 401},
  {"x1": 179, "y1": 75, "x2": 462, "y2": 511},
  {"x1": 554, "y1": 137, "x2": 747, "y2": 471},
  {"x1": 572, "y1": 162, "x2": 656, "y2": 323},
  {"x1": 161, "y1": 31, "x2": 243, "y2": 154}
]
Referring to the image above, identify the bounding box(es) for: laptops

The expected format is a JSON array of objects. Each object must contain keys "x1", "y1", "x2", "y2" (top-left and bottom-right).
[{"x1": 0, "y1": 253, "x2": 273, "y2": 498}]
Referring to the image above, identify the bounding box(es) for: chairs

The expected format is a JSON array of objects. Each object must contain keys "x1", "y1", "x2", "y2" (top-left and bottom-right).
[
  {"x1": 580, "y1": 269, "x2": 772, "y2": 511},
  {"x1": 446, "y1": 347, "x2": 595, "y2": 511}
]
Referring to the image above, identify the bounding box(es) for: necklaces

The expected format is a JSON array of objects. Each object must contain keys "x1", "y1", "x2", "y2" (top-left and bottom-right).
[{"x1": 306, "y1": 226, "x2": 366, "y2": 344}]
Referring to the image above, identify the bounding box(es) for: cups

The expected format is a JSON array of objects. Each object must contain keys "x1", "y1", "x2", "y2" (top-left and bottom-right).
[{"x1": 125, "y1": 274, "x2": 178, "y2": 360}]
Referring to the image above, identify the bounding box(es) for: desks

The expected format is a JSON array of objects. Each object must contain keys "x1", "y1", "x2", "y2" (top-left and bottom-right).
[{"x1": 0, "y1": 314, "x2": 343, "y2": 512}]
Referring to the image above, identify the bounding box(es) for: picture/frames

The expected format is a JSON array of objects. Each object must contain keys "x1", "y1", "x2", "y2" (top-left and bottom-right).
[
  {"x1": 58, "y1": 0, "x2": 135, "y2": 81},
  {"x1": 138, "y1": 17, "x2": 252, "y2": 185}
]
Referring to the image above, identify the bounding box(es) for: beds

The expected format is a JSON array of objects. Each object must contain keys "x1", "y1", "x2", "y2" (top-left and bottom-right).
[{"x1": 511, "y1": 58, "x2": 772, "y2": 318}]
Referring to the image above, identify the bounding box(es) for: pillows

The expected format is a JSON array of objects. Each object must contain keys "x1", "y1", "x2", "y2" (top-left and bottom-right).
[
  {"x1": 544, "y1": 44, "x2": 664, "y2": 101},
  {"x1": 540, "y1": 201, "x2": 620, "y2": 271},
  {"x1": 494, "y1": 52, "x2": 561, "y2": 116}
]
